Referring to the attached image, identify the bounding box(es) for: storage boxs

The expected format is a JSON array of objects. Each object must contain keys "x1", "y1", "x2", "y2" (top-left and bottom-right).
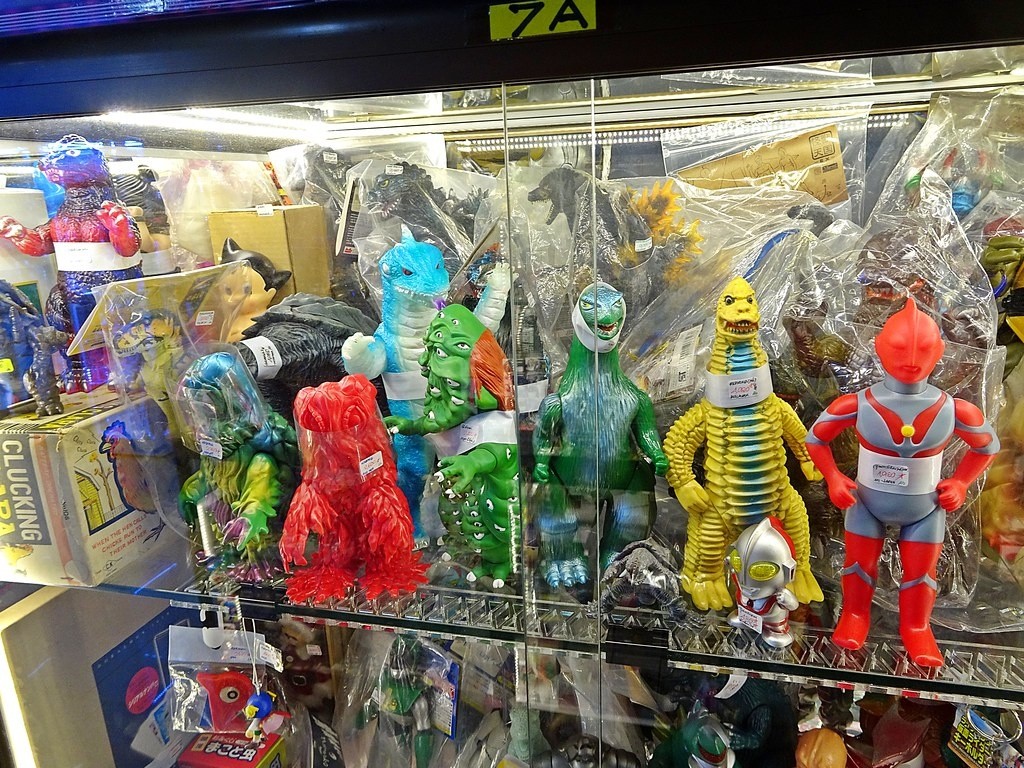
[
  {"x1": 208, "y1": 201, "x2": 335, "y2": 309},
  {"x1": 0, "y1": 379, "x2": 201, "y2": 589},
  {"x1": 179, "y1": 725, "x2": 290, "y2": 768}
]
